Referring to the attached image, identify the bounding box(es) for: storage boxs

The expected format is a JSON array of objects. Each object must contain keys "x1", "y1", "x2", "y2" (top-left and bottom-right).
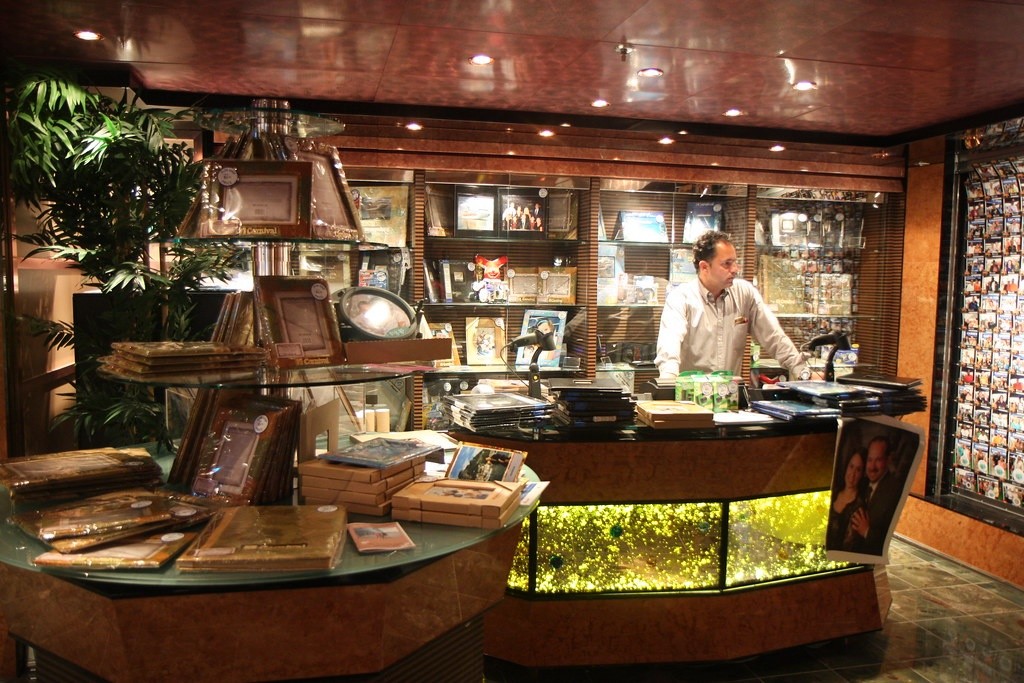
[{"x1": 438, "y1": 259, "x2": 452, "y2": 303}]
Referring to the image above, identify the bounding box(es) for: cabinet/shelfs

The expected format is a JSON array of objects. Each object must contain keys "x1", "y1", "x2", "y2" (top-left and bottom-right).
[{"x1": 341, "y1": 148, "x2": 904, "y2": 400}]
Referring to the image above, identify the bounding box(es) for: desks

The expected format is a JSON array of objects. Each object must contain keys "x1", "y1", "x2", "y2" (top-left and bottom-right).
[{"x1": 0, "y1": 442, "x2": 539, "y2": 683}]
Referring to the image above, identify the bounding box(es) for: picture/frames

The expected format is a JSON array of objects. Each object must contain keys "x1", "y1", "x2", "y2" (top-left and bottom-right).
[
  {"x1": 178, "y1": 129, "x2": 365, "y2": 243},
  {"x1": 537, "y1": 267, "x2": 578, "y2": 306},
  {"x1": 254, "y1": 275, "x2": 344, "y2": 369},
  {"x1": 498, "y1": 187, "x2": 547, "y2": 238},
  {"x1": 341, "y1": 287, "x2": 418, "y2": 341},
  {"x1": 636, "y1": 399, "x2": 716, "y2": 428},
  {"x1": 167, "y1": 386, "x2": 302, "y2": 504},
  {"x1": 424, "y1": 185, "x2": 454, "y2": 238},
  {"x1": 299, "y1": 252, "x2": 351, "y2": 295},
  {"x1": 298, "y1": 446, "x2": 427, "y2": 517},
  {"x1": 466, "y1": 317, "x2": 507, "y2": 365},
  {"x1": 547, "y1": 190, "x2": 578, "y2": 240},
  {"x1": 454, "y1": 185, "x2": 499, "y2": 238},
  {"x1": 96, "y1": 292, "x2": 271, "y2": 384},
  {"x1": 682, "y1": 200, "x2": 722, "y2": 244},
  {"x1": 515, "y1": 309, "x2": 567, "y2": 367},
  {"x1": 391, "y1": 475, "x2": 526, "y2": 529},
  {"x1": 0, "y1": 446, "x2": 347, "y2": 572},
  {"x1": 504, "y1": 266, "x2": 537, "y2": 304},
  {"x1": 618, "y1": 211, "x2": 669, "y2": 243},
  {"x1": 427, "y1": 323, "x2": 461, "y2": 366}
]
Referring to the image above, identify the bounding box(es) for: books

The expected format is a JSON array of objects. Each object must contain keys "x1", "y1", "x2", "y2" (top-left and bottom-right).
[{"x1": 437, "y1": 372, "x2": 927, "y2": 438}]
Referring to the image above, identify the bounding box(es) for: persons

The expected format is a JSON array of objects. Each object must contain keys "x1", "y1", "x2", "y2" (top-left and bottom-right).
[
  {"x1": 849, "y1": 435, "x2": 901, "y2": 556},
  {"x1": 485, "y1": 454, "x2": 511, "y2": 468},
  {"x1": 826, "y1": 448, "x2": 869, "y2": 551},
  {"x1": 502, "y1": 203, "x2": 543, "y2": 232},
  {"x1": 522, "y1": 320, "x2": 557, "y2": 361},
  {"x1": 656, "y1": 229, "x2": 824, "y2": 382},
  {"x1": 954, "y1": 153, "x2": 1024, "y2": 508}
]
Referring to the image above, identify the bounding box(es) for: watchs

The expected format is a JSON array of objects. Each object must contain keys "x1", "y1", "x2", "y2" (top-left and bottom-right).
[{"x1": 797, "y1": 367, "x2": 812, "y2": 380}]
